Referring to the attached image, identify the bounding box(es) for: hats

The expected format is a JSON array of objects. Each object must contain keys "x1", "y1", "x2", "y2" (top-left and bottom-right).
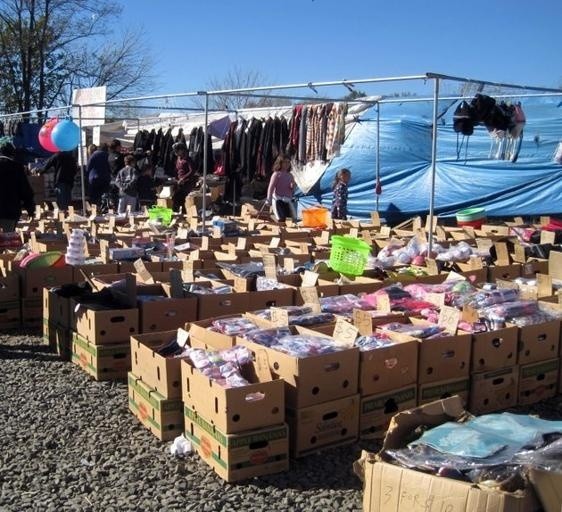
[
  {"x1": 172, "y1": 142, "x2": 185, "y2": 152},
  {"x1": 453, "y1": 93, "x2": 526, "y2": 139}
]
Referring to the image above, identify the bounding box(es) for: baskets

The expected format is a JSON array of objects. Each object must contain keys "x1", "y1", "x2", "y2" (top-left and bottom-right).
[
  {"x1": 147, "y1": 207, "x2": 173, "y2": 225},
  {"x1": 329, "y1": 235, "x2": 373, "y2": 276},
  {"x1": 301, "y1": 209, "x2": 329, "y2": 228}
]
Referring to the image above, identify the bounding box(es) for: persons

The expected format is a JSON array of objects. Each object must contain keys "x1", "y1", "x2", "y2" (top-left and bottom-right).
[
  {"x1": 33, "y1": 139, "x2": 195, "y2": 215},
  {"x1": 265, "y1": 154, "x2": 298, "y2": 222},
  {"x1": 0, "y1": 141, "x2": 35, "y2": 234},
  {"x1": 331, "y1": 168, "x2": 350, "y2": 220}
]
